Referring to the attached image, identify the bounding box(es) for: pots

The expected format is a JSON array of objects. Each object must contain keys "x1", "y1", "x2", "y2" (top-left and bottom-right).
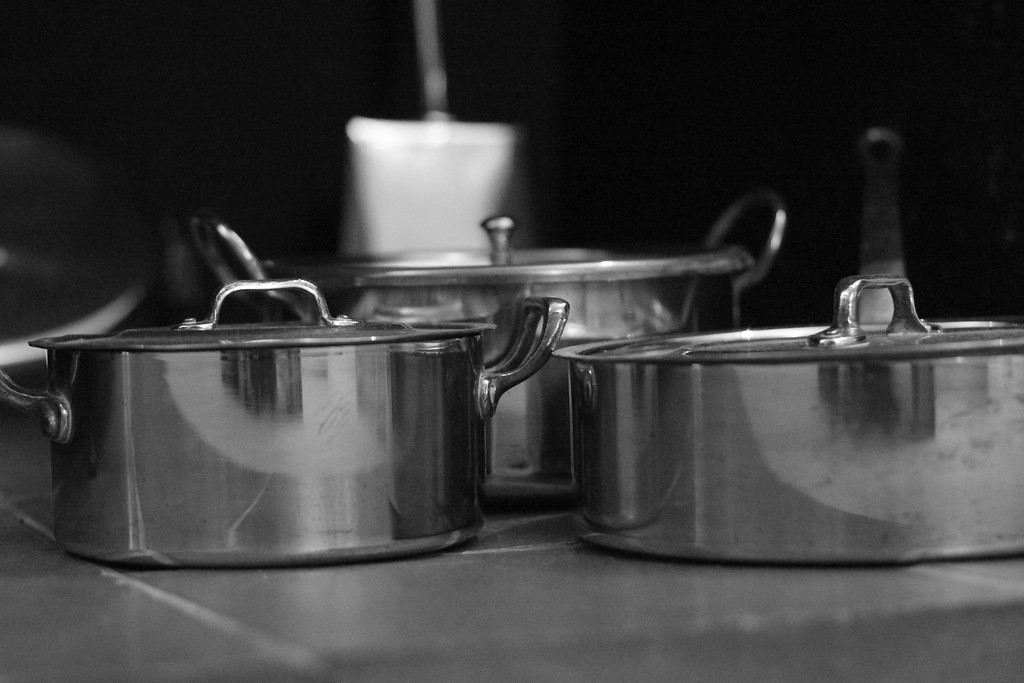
[
  {"x1": 179, "y1": 184, "x2": 790, "y2": 515},
  {"x1": 551, "y1": 274, "x2": 1024, "y2": 564},
  {"x1": 0, "y1": 277, "x2": 569, "y2": 569}
]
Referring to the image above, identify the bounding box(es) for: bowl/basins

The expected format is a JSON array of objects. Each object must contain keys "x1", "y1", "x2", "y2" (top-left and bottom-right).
[{"x1": 0, "y1": 120, "x2": 159, "y2": 371}]
